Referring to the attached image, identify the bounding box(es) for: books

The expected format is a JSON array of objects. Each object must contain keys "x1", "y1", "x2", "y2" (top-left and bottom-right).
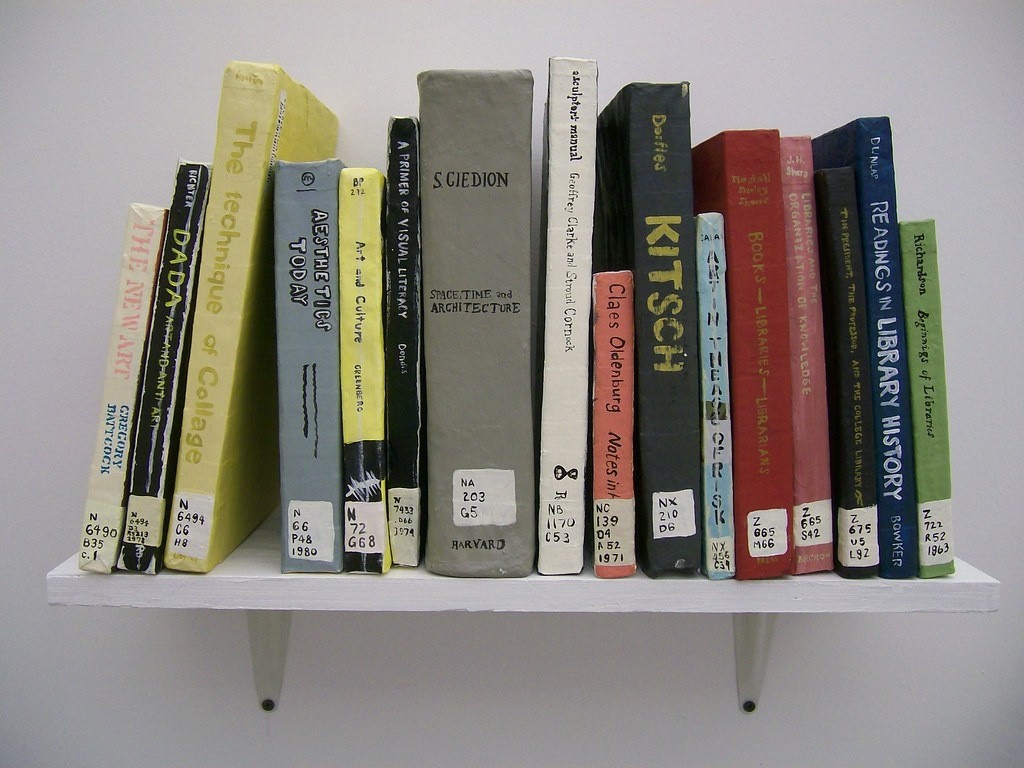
[
  {"x1": 383, "y1": 115, "x2": 425, "y2": 567},
  {"x1": 273, "y1": 159, "x2": 347, "y2": 573},
  {"x1": 898, "y1": 218, "x2": 956, "y2": 579},
  {"x1": 78, "y1": 204, "x2": 169, "y2": 575},
  {"x1": 811, "y1": 116, "x2": 918, "y2": 578},
  {"x1": 591, "y1": 270, "x2": 636, "y2": 579},
  {"x1": 163, "y1": 62, "x2": 339, "y2": 573},
  {"x1": 779, "y1": 135, "x2": 833, "y2": 574},
  {"x1": 816, "y1": 167, "x2": 881, "y2": 579},
  {"x1": 338, "y1": 166, "x2": 392, "y2": 575},
  {"x1": 690, "y1": 130, "x2": 795, "y2": 580},
  {"x1": 695, "y1": 212, "x2": 736, "y2": 581},
  {"x1": 590, "y1": 82, "x2": 701, "y2": 579},
  {"x1": 117, "y1": 159, "x2": 213, "y2": 574},
  {"x1": 536, "y1": 56, "x2": 599, "y2": 573},
  {"x1": 416, "y1": 70, "x2": 535, "y2": 578}
]
